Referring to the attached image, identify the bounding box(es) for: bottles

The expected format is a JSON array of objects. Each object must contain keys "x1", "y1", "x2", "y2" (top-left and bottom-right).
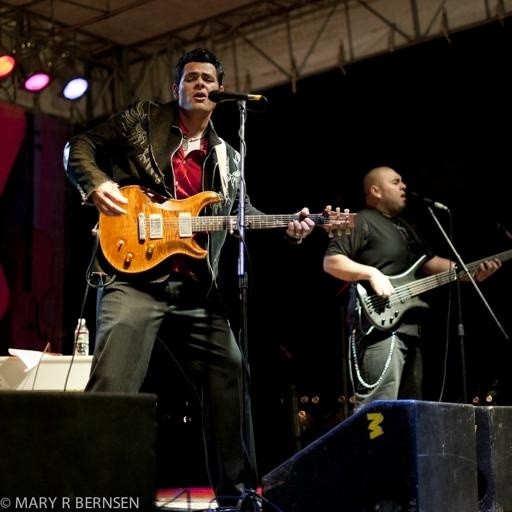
[{"x1": 74, "y1": 318, "x2": 89, "y2": 357}]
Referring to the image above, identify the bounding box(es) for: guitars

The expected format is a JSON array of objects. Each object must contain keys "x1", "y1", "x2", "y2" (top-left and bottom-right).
[
  {"x1": 357, "y1": 251, "x2": 512, "y2": 331},
  {"x1": 97, "y1": 184, "x2": 356, "y2": 283}
]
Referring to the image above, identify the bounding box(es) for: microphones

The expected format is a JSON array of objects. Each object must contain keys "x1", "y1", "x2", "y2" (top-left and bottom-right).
[
  {"x1": 409, "y1": 191, "x2": 448, "y2": 210},
  {"x1": 209, "y1": 90, "x2": 264, "y2": 102}
]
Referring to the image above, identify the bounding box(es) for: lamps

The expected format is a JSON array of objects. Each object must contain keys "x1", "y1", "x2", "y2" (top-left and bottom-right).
[
  {"x1": 15, "y1": 47, "x2": 51, "y2": 94},
  {"x1": 49, "y1": 48, "x2": 91, "y2": 101},
  {"x1": 0, "y1": 44, "x2": 17, "y2": 76}
]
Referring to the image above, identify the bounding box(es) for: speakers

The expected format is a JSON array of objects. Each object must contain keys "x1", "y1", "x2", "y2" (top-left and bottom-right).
[
  {"x1": 239, "y1": 399, "x2": 480, "y2": 512},
  {"x1": 0, "y1": 389, "x2": 158, "y2": 512},
  {"x1": 471, "y1": 406, "x2": 512, "y2": 512}
]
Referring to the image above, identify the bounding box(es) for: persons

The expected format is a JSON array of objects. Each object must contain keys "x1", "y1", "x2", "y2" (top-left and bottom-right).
[
  {"x1": 64, "y1": 47, "x2": 316, "y2": 512},
  {"x1": 323, "y1": 166, "x2": 503, "y2": 405}
]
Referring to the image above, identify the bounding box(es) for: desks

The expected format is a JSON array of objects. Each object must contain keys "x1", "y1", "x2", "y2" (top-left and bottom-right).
[{"x1": 0, "y1": 355, "x2": 92, "y2": 392}]
